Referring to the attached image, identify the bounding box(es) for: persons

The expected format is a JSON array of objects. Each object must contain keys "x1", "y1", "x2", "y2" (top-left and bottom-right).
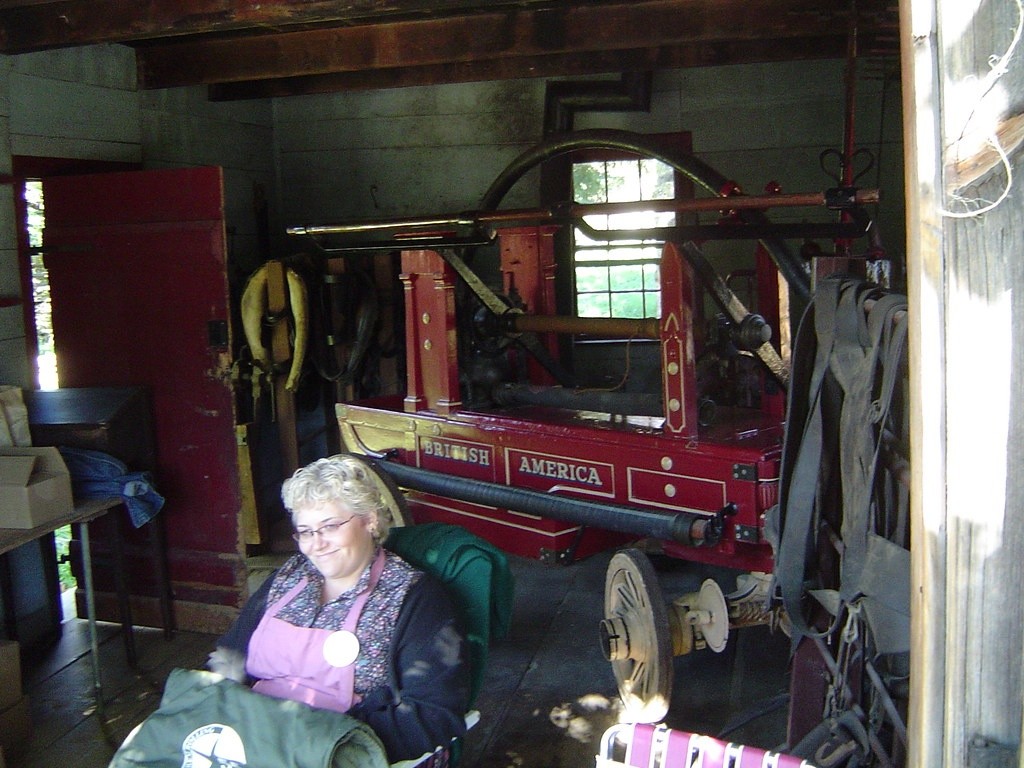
[{"x1": 199, "y1": 454, "x2": 466, "y2": 764}]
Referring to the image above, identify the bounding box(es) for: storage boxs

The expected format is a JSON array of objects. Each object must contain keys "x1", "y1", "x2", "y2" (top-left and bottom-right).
[
  {"x1": 0, "y1": 689, "x2": 33, "y2": 752},
  {"x1": 0, "y1": 446, "x2": 74, "y2": 528},
  {"x1": 0, "y1": 640, "x2": 23, "y2": 711}
]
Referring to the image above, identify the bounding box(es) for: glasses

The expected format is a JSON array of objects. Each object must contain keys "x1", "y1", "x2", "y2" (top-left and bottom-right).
[{"x1": 291, "y1": 515, "x2": 357, "y2": 542}]
